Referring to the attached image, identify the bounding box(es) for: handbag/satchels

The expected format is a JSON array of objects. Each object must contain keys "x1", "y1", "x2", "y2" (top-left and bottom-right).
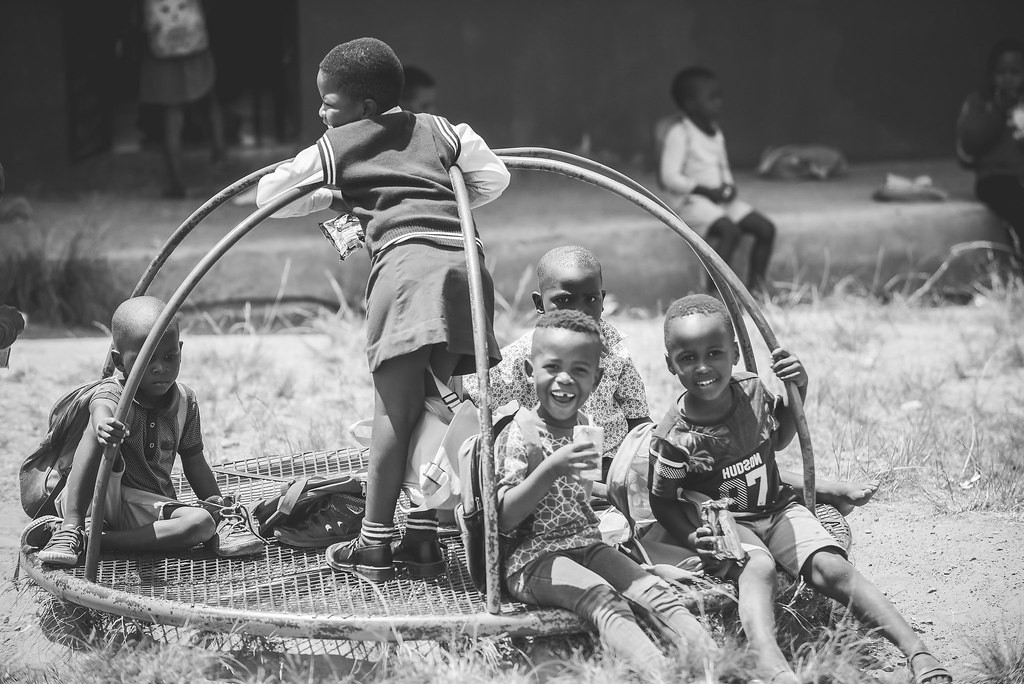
[{"x1": 253, "y1": 475, "x2": 367, "y2": 538}]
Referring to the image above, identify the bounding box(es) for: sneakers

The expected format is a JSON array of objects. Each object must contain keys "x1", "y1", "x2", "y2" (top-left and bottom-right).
[
  {"x1": 274, "y1": 493, "x2": 365, "y2": 547},
  {"x1": 201, "y1": 495, "x2": 263, "y2": 556}
]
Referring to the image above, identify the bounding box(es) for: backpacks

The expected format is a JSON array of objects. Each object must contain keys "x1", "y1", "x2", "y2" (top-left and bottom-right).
[
  {"x1": 348, "y1": 363, "x2": 480, "y2": 510},
  {"x1": 455, "y1": 407, "x2": 520, "y2": 591},
  {"x1": 19, "y1": 381, "x2": 101, "y2": 519}
]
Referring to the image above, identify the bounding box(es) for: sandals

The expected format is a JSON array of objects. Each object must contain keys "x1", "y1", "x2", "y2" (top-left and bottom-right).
[{"x1": 907, "y1": 652, "x2": 952, "y2": 684}]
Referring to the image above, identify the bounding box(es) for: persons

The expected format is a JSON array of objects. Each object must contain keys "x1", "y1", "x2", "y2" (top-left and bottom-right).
[
  {"x1": 955, "y1": 37, "x2": 1024, "y2": 260},
  {"x1": 143, "y1": 0, "x2": 227, "y2": 197},
  {"x1": 256, "y1": 39, "x2": 511, "y2": 583},
  {"x1": 461, "y1": 247, "x2": 752, "y2": 684},
  {"x1": 39, "y1": 297, "x2": 222, "y2": 564},
  {"x1": 647, "y1": 294, "x2": 951, "y2": 684},
  {"x1": 649, "y1": 67, "x2": 786, "y2": 298}
]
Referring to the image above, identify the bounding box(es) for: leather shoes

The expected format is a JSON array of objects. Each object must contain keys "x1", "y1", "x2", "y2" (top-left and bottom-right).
[
  {"x1": 391, "y1": 539, "x2": 445, "y2": 576},
  {"x1": 326, "y1": 537, "x2": 395, "y2": 580}
]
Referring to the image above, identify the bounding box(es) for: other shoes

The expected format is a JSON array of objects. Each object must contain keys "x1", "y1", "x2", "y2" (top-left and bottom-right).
[{"x1": 21, "y1": 515, "x2": 88, "y2": 564}]
